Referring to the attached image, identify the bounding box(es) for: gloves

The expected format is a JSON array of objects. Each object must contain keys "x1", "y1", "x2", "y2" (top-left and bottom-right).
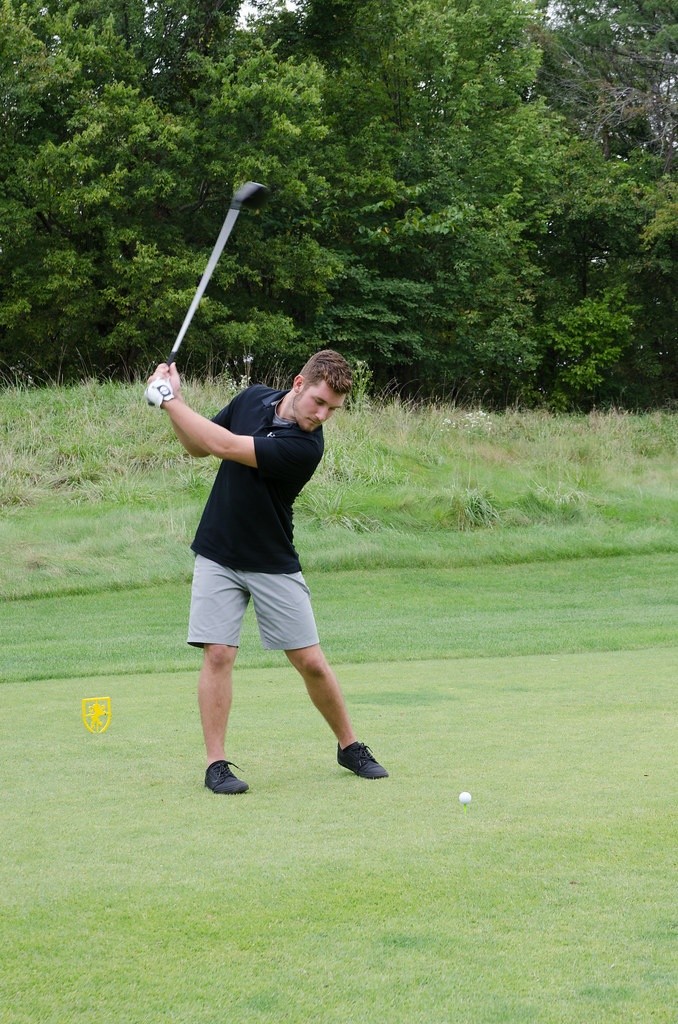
[{"x1": 145, "y1": 378, "x2": 175, "y2": 408}]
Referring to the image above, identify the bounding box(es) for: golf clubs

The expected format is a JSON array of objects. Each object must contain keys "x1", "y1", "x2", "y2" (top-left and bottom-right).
[{"x1": 146, "y1": 183, "x2": 265, "y2": 408}]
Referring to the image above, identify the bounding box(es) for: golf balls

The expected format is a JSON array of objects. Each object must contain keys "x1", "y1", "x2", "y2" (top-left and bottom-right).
[{"x1": 458, "y1": 791, "x2": 471, "y2": 804}]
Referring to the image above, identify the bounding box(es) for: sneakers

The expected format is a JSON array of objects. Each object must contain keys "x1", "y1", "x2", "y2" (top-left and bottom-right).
[
  {"x1": 337, "y1": 741, "x2": 388, "y2": 777},
  {"x1": 204, "y1": 760, "x2": 249, "y2": 793}
]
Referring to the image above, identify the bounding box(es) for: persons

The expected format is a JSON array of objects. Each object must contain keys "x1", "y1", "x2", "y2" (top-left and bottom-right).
[{"x1": 141, "y1": 350, "x2": 388, "y2": 795}]
